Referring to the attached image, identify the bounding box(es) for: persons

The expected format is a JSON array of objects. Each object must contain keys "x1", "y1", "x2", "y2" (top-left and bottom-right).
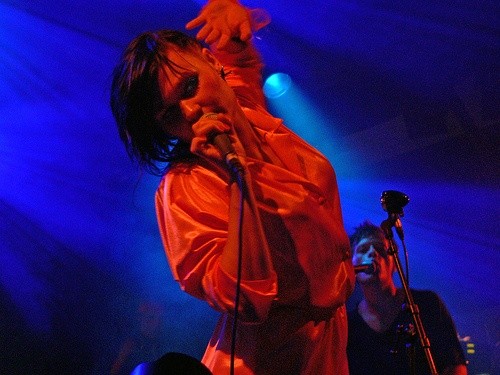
[
  {"x1": 348, "y1": 220, "x2": 467, "y2": 375},
  {"x1": 109, "y1": 0, "x2": 356, "y2": 375}
]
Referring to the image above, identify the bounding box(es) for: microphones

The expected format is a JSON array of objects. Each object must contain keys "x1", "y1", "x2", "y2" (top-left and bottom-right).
[
  {"x1": 353, "y1": 263, "x2": 376, "y2": 274},
  {"x1": 197, "y1": 112, "x2": 244, "y2": 188}
]
